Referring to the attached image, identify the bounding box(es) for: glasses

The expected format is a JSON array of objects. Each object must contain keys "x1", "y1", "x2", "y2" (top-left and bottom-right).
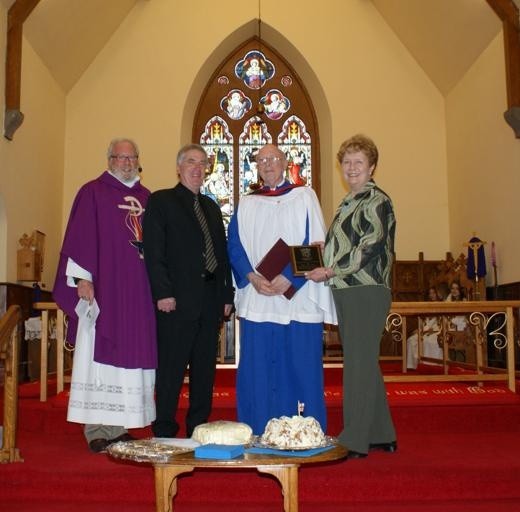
[
  {"x1": 111, "y1": 153, "x2": 139, "y2": 162},
  {"x1": 259, "y1": 157, "x2": 281, "y2": 164}
]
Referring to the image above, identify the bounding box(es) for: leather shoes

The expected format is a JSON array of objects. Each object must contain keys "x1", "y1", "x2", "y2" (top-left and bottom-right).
[
  {"x1": 350, "y1": 442, "x2": 397, "y2": 459},
  {"x1": 89, "y1": 434, "x2": 136, "y2": 454}
]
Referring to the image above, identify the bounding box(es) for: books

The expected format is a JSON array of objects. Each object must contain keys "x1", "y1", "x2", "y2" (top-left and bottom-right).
[{"x1": 253, "y1": 236, "x2": 309, "y2": 301}]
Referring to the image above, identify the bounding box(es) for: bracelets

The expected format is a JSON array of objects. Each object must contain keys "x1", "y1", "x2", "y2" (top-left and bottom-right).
[{"x1": 325, "y1": 267, "x2": 330, "y2": 280}]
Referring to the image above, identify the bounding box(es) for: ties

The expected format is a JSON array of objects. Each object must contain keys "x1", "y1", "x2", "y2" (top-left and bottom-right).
[{"x1": 194, "y1": 197, "x2": 218, "y2": 274}]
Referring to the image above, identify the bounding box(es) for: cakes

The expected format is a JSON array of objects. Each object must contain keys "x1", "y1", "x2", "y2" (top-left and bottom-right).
[
  {"x1": 191, "y1": 420, "x2": 254, "y2": 445},
  {"x1": 259, "y1": 399, "x2": 327, "y2": 450}
]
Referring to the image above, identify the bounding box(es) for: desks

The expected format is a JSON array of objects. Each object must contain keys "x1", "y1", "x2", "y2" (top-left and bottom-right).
[{"x1": 106, "y1": 436, "x2": 348, "y2": 511}]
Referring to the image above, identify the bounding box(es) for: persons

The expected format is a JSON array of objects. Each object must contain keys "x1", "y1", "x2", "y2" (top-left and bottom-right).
[
  {"x1": 423, "y1": 278, "x2": 470, "y2": 365},
  {"x1": 52, "y1": 137, "x2": 161, "y2": 455},
  {"x1": 224, "y1": 142, "x2": 340, "y2": 439},
  {"x1": 405, "y1": 284, "x2": 442, "y2": 370},
  {"x1": 141, "y1": 143, "x2": 236, "y2": 441},
  {"x1": 301, "y1": 132, "x2": 398, "y2": 461}
]
